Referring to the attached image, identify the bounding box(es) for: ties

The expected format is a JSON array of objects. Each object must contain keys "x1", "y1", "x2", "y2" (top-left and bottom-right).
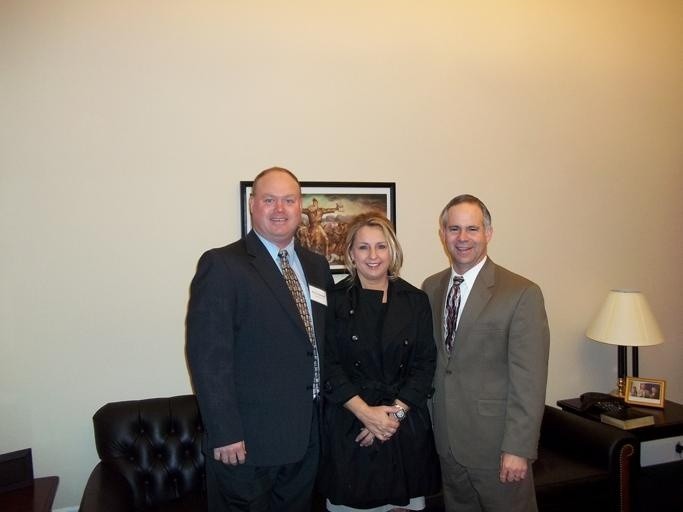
[
  {"x1": 276, "y1": 249, "x2": 323, "y2": 402},
  {"x1": 442, "y1": 274, "x2": 466, "y2": 349}
]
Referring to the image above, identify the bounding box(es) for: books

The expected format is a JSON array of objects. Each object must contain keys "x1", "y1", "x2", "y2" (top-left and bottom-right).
[{"x1": 599, "y1": 408, "x2": 656, "y2": 430}]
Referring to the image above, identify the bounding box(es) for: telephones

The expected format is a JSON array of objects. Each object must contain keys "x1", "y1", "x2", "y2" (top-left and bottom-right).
[{"x1": 580, "y1": 392, "x2": 625, "y2": 410}]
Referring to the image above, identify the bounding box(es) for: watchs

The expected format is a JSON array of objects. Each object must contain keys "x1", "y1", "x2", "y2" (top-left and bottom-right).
[{"x1": 391, "y1": 401, "x2": 407, "y2": 422}]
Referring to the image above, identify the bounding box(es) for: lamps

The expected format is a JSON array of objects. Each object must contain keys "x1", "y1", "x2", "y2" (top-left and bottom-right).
[{"x1": 587, "y1": 289, "x2": 664, "y2": 398}]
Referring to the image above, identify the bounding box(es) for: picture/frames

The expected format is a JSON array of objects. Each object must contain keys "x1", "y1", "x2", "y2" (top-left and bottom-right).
[
  {"x1": 624, "y1": 377, "x2": 665, "y2": 407},
  {"x1": 240, "y1": 181, "x2": 396, "y2": 274}
]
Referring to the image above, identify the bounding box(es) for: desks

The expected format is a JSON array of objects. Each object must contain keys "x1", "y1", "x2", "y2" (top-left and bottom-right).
[
  {"x1": 557, "y1": 392, "x2": 683, "y2": 511},
  {"x1": 0, "y1": 476, "x2": 59, "y2": 512}
]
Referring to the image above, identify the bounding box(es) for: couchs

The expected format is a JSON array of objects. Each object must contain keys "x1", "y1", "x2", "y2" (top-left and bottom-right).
[{"x1": 79, "y1": 394, "x2": 640, "y2": 512}]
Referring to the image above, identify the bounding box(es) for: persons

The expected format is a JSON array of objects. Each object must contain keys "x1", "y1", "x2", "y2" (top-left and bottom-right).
[
  {"x1": 419, "y1": 193, "x2": 551, "y2": 511},
  {"x1": 318, "y1": 211, "x2": 444, "y2": 512},
  {"x1": 631, "y1": 383, "x2": 657, "y2": 398},
  {"x1": 296, "y1": 198, "x2": 349, "y2": 263},
  {"x1": 185, "y1": 167, "x2": 334, "y2": 512}
]
